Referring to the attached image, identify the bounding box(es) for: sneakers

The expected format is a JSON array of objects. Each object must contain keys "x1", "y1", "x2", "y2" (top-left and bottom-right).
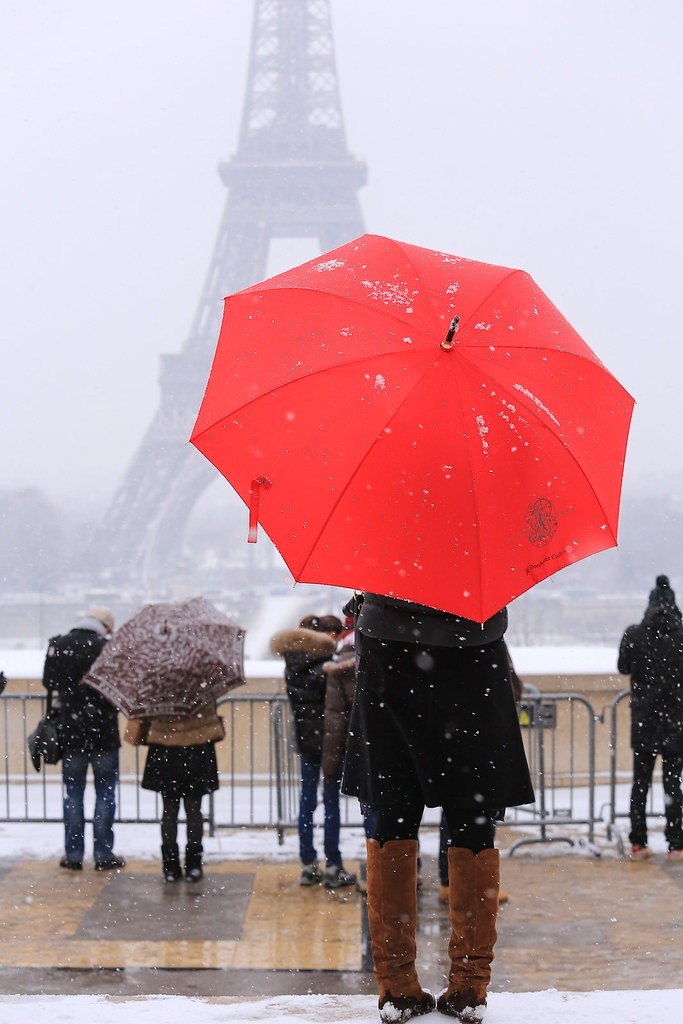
[
  {"x1": 300, "y1": 859, "x2": 325, "y2": 886},
  {"x1": 324, "y1": 864, "x2": 357, "y2": 887}
]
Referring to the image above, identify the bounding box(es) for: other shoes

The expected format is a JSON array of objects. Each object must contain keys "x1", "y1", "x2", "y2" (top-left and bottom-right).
[
  {"x1": 630, "y1": 844, "x2": 652, "y2": 861},
  {"x1": 666, "y1": 848, "x2": 682, "y2": 861},
  {"x1": 416, "y1": 857, "x2": 424, "y2": 888},
  {"x1": 60, "y1": 856, "x2": 82, "y2": 870},
  {"x1": 357, "y1": 862, "x2": 367, "y2": 894},
  {"x1": 497, "y1": 892, "x2": 506, "y2": 903},
  {"x1": 94, "y1": 856, "x2": 125, "y2": 871},
  {"x1": 438, "y1": 886, "x2": 451, "y2": 903}
]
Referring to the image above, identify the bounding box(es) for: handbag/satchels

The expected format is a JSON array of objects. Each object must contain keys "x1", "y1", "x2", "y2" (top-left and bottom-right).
[
  {"x1": 27, "y1": 704, "x2": 62, "y2": 772},
  {"x1": 123, "y1": 717, "x2": 149, "y2": 746}
]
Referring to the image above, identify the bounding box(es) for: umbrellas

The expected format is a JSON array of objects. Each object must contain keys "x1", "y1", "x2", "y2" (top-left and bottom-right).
[
  {"x1": 81, "y1": 595, "x2": 248, "y2": 722},
  {"x1": 184, "y1": 233, "x2": 637, "y2": 629}
]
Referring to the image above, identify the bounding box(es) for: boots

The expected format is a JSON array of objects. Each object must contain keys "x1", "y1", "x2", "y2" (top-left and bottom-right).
[
  {"x1": 437, "y1": 848, "x2": 500, "y2": 1024},
  {"x1": 184, "y1": 845, "x2": 203, "y2": 881},
  {"x1": 364, "y1": 838, "x2": 436, "y2": 1024},
  {"x1": 162, "y1": 843, "x2": 183, "y2": 881}
]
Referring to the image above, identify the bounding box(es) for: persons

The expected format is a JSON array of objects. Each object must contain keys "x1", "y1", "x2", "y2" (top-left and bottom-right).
[
  {"x1": 617, "y1": 574, "x2": 683, "y2": 861},
  {"x1": 340, "y1": 584, "x2": 536, "y2": 1024},
  {"x1": 142, "y1": 700, "x2": 226, "y2": 881},
  {"x1": 41, "y1": 607, "x2": 126, "y2": 871},
  {"x1": 437, "y1": 808, "x2": 509, "y2": 905},
  {"x1": 270, "y1": 614, "x2": 358, "y2": 889},
  {"x1": 319, "y1": 630, "x2": 424, "y2": 899}
]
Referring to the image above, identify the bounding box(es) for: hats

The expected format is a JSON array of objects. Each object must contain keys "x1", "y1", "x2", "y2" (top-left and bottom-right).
[
  {"x1": 648, "y1": 575, "x2": 675, "y2": 605},
  {"x1": 87, "y1": 605, "x2": 114, "y2": 633},
  {"x1": 342, "y1": 594, "x2": 365, "y2": 617}
]
